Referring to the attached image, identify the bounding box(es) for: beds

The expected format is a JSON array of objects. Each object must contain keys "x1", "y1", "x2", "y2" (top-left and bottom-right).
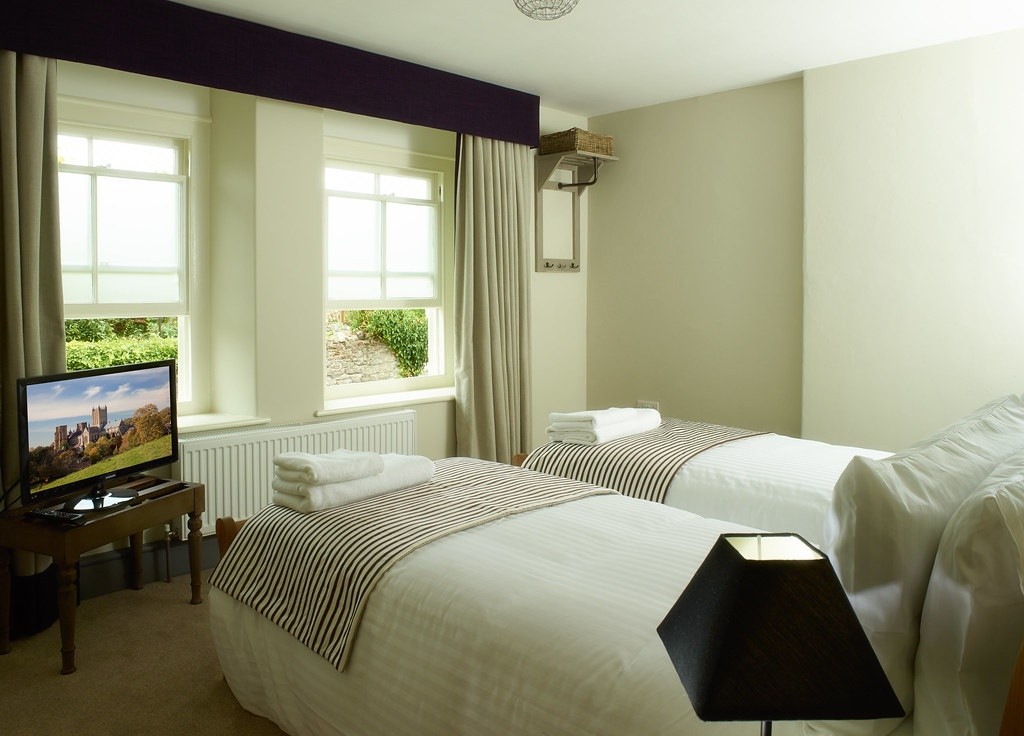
[
  {"x1": 515, "y1": 420, "x2": 894, "y2": 543},
  {"x1": 205, "y1": 458, "x2": 1024, "y2": 736}
]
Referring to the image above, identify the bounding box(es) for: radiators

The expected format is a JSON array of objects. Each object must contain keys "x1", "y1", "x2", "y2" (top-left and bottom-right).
[{"x1": 173, "y1": 409, "x2": 416, "y2": 541}]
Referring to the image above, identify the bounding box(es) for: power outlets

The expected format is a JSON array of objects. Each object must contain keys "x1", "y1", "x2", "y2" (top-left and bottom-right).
[{"x1": 638, "y1": 400, "x2": 659, "y2": 412}]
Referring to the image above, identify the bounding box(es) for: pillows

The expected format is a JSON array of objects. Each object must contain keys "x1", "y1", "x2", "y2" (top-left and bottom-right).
[{"x1": 802, "y1": 392, "x2": 1024, "y2": 736}]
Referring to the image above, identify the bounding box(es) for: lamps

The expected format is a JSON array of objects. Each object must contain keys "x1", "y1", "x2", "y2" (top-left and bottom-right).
[
  {"x1": 656, "y1": 534, "x2": 906, "y2": 736},
  {"x1": 513, "y1": 0, "x2": 579, "y2": 22}
]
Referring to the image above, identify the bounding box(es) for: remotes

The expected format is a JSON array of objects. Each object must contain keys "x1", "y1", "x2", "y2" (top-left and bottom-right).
[{"x1": 25, "y1": 508, "x2": 87, "y2": 526}]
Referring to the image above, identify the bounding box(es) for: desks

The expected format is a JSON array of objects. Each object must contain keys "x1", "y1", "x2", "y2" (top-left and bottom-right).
[{"x1": 0, "y1": 475, "x2": 204, "y2": 675}]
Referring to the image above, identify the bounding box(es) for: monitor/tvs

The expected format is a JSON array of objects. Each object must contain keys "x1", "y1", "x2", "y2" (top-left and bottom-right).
[{"x1": 16, "y1": 359, "x2": 179, "y2": 514}]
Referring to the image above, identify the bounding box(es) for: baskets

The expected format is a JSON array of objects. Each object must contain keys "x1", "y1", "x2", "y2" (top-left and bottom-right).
[{"x1": 539, "y1": 126, "x2": 614, "y2": 157}]
